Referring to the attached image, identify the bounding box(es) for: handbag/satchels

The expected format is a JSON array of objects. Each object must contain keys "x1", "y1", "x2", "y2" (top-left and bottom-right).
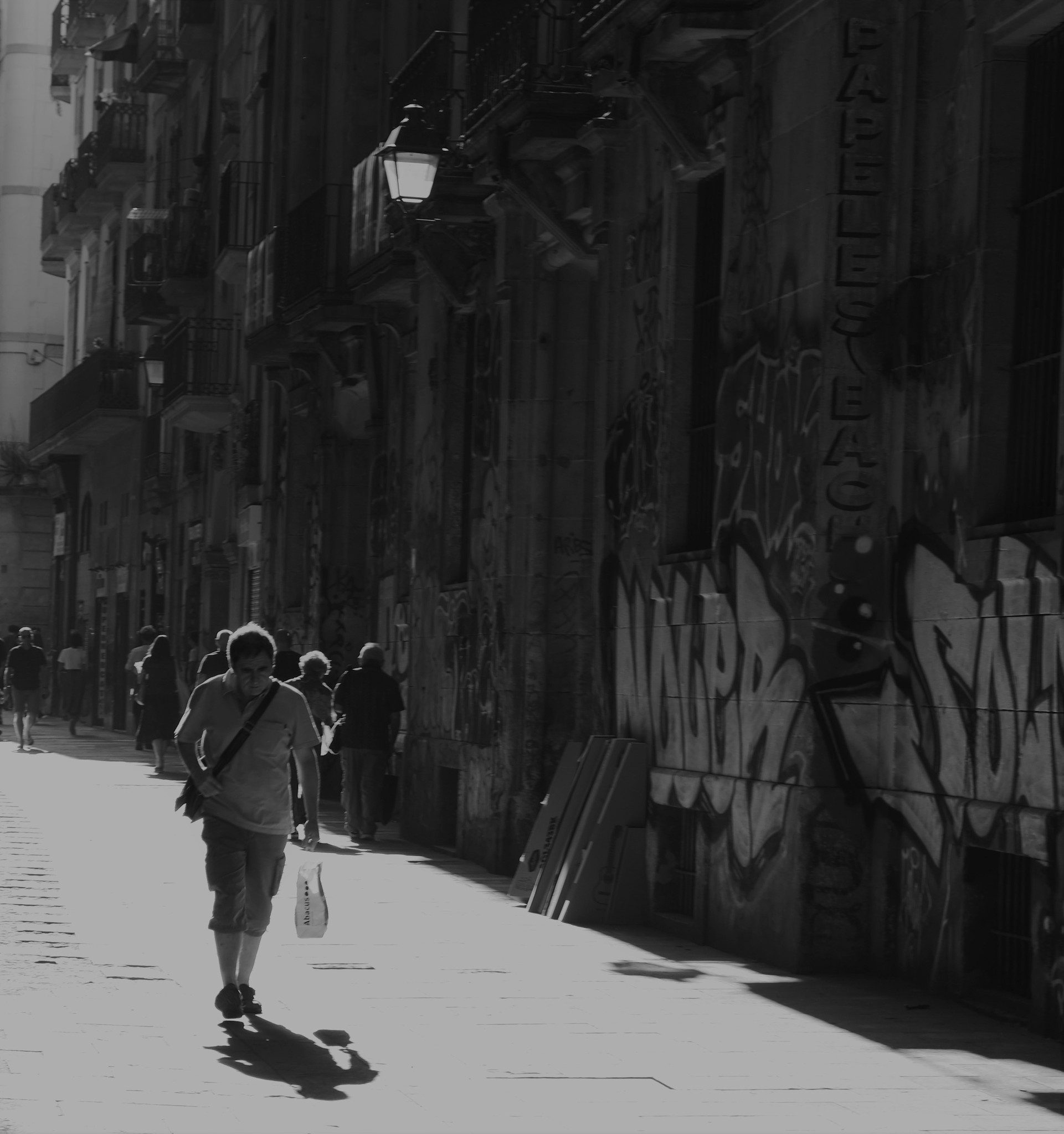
[
  {"x1": 327, "y1": 723, "x2": 342, "y2": 755},
  {"x1": 295, "y1": 848, "x2": 328, "y2": 938},
  {"x1": 174, "y1": 769, "x2": 209, "y2": 822}
]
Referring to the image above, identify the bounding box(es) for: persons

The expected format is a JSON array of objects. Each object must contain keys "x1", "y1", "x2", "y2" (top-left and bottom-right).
[
  {"x1": 56, "y1": 633, "x2": 93, "y2": 736},
  {"x1": 123, "y1": 624, "x2": 162, "y2": 752},
  {"x1": 174, "y1": 622, "x2": 319, "y2": 1021},
  {"x1": 331, "y1": 642, "x2": 407, "y2": 838},
  {"x1": 131, "y1": 633, "x2": 175, "y2": 773},
  {"x1": 267, "y1": 627, "x2": 303, "y2": 825},
  {"x1": 0, "y1": 622, "x2": 53, "y2": 748},
  {"x1": 283, "y1": 650, "x2": 332, "y2": 841},
  {"x1": 195, "y1": 628, "x2": 234, "y2": 754}
]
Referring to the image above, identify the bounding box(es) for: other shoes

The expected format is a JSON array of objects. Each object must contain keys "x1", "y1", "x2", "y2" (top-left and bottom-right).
[
  {"x1": 214, "y1": 984, "x2": 243, "y2": 1020},
  {"x1": 22, "y1": 733, "x2": 34, "y2": 746},
  {"x1": 69, "y1": 725, "x2": 76, "y2": 737},
  {"x1": 240, "y1": 983, "x2": 262, "y2": 1015},
  {"x1": 348, "y1": 830, "x2": 373, "y2": 840},
  {"x1": 291, "y1": 829, "x2": 299, "y2": 839},
  {"x1": 135, "y1": 744, "x2": 143, "y2": 750},
  {"x1": 154, "y1": 764, "x2": 165, "y2": 773},
  {"x1": 18, "y1": 744, "x2": 23, "y2": 750},
  {"x1": 145, "y1": 744, "x2": 153, "y2": 751}
]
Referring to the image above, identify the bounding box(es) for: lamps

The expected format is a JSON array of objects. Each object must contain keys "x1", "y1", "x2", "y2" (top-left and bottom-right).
[
  {"x1": 373, "y1": 103, "x2": 497, "y2": 292},
  {"x1": 138, "y1": 334, "x2": 168, "y2": 405}
]
